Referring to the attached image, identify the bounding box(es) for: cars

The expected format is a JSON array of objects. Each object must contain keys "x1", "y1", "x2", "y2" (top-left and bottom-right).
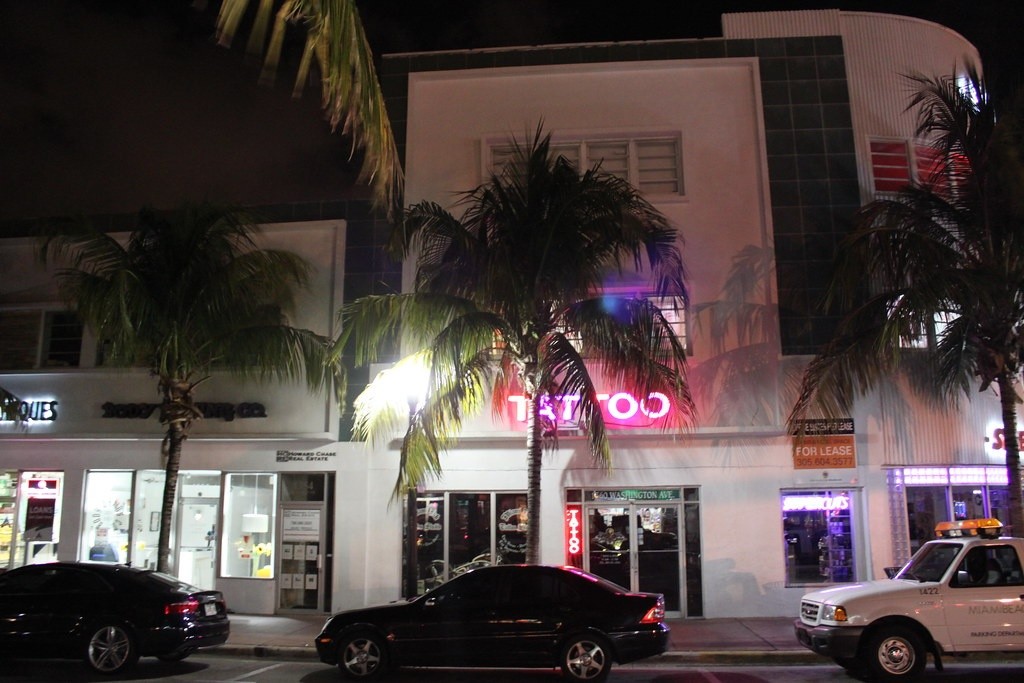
[
  {"x1": 0, "y1": 562, "x2": 231, "y2": 679},
  {"x1": 315, "y1": 565, "x2": 672, "y2": 683}
]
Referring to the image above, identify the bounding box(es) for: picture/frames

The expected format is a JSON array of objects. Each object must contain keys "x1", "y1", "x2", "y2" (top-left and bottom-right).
[{"x1": 150, "y1": 512, "x2": 159, "y2": 531}]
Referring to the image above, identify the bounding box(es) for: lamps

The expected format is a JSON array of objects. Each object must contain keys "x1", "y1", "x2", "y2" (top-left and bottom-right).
[{"x1": 242, "y1": 475, "x2": 268, "y2": 533}]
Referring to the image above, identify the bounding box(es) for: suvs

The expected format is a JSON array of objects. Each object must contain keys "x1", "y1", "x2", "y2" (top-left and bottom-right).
[{"x1": 794, "y1": 534, "x2": 1024, "y2": 683}]
